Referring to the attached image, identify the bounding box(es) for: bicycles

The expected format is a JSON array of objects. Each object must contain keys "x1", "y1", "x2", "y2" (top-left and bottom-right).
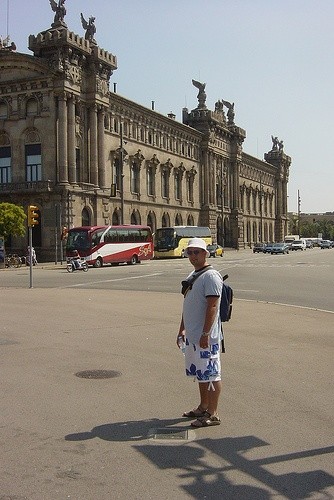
[{"x1": 5, "y1": 252, "x2": 23, "y2": 268}]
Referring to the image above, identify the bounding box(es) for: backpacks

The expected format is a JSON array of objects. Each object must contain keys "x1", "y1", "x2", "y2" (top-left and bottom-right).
[{"x1": 219, "y1": 274, "x2": 233, "y2": 322}]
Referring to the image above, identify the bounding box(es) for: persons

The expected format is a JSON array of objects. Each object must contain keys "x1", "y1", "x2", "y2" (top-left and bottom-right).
[
  {"x1": 27, "y1": 246, "x2": 38, "y2": 266},
  {"x1": 176, "y1": 238, "x2": 222, "y2": 427},
  {"x1": 73, "y1": 250, "x2": 81, "y2": 268}
]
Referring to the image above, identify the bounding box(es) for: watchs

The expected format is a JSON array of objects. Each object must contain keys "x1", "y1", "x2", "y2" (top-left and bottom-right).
[{"x1": 202, "y1": 331, "x2": 209, "y2": 336}]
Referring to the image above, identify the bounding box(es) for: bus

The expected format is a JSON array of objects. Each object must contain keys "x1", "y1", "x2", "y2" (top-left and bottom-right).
[
  {"x1": 65, "y1": 225, "x2": 155, "y2": 268},
  {"x1": 151, "y1": 226, "x2": 212, "y2": 259}
]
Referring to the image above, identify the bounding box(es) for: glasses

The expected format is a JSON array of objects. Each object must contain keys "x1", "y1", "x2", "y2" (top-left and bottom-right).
[{"x1": 187, "y1": 249, "x2": 203, "y2": 254}]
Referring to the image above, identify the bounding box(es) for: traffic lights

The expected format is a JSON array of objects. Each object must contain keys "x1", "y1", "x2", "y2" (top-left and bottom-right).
[{"x1": 27, "y1": 206, "x2": 39, "y2": 226}]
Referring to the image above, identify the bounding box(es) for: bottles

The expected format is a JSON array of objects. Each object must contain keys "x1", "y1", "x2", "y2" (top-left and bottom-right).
[{"x1": 178, "y1": 335, "x2": 185, "y2": 356}]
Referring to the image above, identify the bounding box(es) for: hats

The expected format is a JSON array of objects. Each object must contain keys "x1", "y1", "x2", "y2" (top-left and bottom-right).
[{"x1": 184, "y1": 238, "x2": 210, "y2": 258}]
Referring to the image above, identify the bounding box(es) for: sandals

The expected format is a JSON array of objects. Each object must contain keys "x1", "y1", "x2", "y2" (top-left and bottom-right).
[
  {"x1": 183, "y1": 404, "x2": 208, "y2": 417},
  {"x1": 192, "y1": 411, "x2": 221, "y2": 427}
]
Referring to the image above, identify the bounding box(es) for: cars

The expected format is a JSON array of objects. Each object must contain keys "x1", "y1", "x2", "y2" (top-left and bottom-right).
[
  {"x1": 205, "y1": 244, "x2": 224, "y2": 258},
  {"x1": 251, "y1": 238, "x2": 334, "y2": 255}
]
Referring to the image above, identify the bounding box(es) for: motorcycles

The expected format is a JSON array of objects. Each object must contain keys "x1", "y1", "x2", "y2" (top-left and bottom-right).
[{"x1": 66, "y1": 257, "x2": 88, "y2": 273}]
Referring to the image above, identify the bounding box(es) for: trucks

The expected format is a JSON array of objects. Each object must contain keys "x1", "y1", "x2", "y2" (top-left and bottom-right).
[{"x1": 285, "y1": 235, "x2": 299, "y2": 246}]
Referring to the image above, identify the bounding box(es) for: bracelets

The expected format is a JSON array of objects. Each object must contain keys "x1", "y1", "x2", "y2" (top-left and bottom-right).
[{"x1": 177, "y1": 334, "x2": 183, "y2": 340}]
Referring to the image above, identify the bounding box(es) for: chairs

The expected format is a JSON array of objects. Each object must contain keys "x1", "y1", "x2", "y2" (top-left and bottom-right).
[{"x1": 109, "y1": 236, "x2": 145, "y2": 241}]
